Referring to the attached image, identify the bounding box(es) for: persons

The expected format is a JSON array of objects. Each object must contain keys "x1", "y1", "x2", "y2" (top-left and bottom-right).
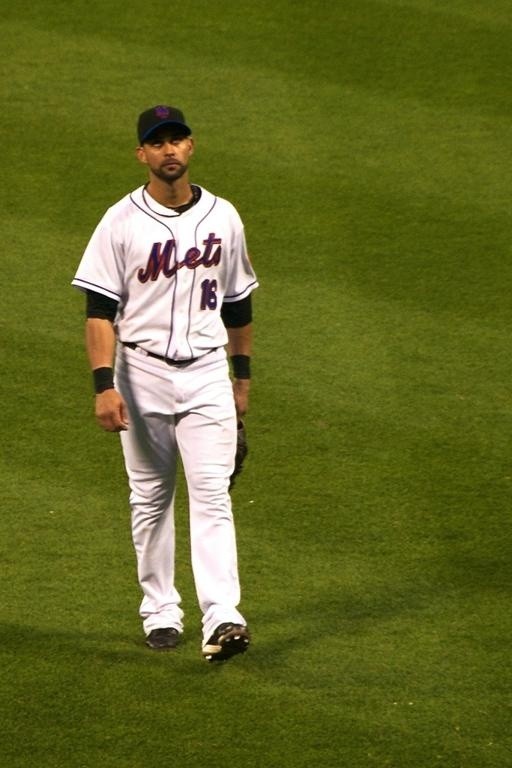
[{"x1": 71, "y1": 104, "x2": 261, "y2": 663}]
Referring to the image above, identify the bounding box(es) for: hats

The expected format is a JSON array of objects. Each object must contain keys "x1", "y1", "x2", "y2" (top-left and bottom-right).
[{"x1": 136, "y1": 105, "x2": 192, "y2": 145}]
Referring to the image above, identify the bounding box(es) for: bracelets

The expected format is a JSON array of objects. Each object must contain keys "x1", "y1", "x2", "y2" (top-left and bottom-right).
[
  {"x1": 91, "y1": 365, "x2": 114, "y2": 394},
  {"x1": 229, "y1": 354, "x2": 250, "y2": 380}
]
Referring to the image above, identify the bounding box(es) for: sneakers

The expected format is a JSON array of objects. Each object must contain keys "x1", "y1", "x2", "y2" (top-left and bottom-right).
[
  {"x1": 201, "y1": 622, "x2": 251, "y2": 665},
  {"x1": 144, "y1": 626, "x2": 180, "y2": 649}
]
